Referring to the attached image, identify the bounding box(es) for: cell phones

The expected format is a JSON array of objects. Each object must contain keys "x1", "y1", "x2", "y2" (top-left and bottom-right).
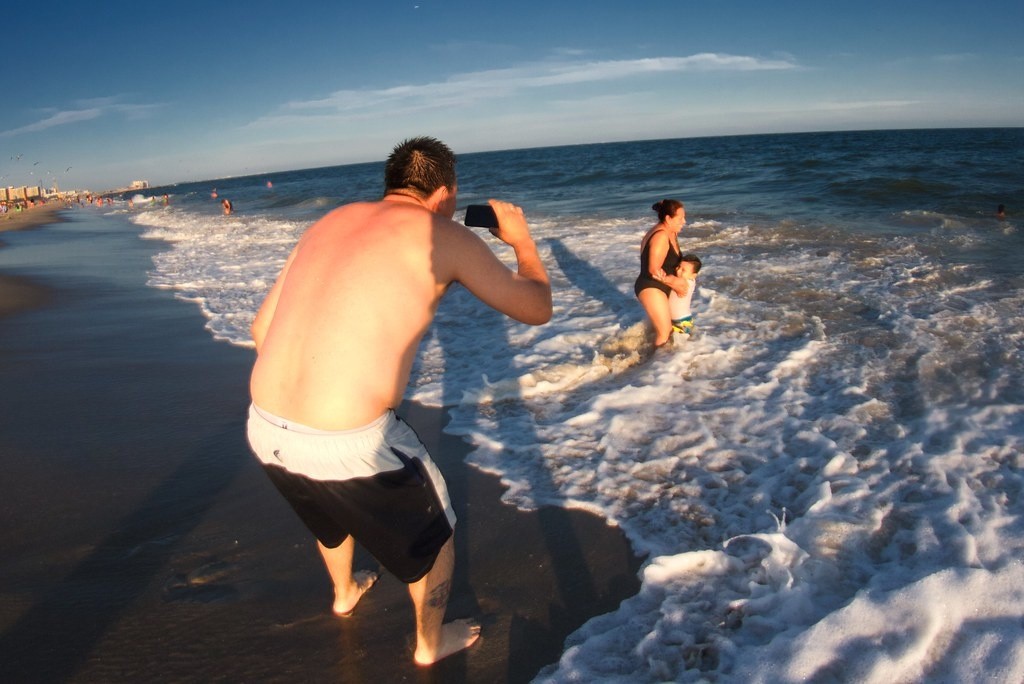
[{"x1": 464, "y1": 205, "x2": 498, "y2": 229}]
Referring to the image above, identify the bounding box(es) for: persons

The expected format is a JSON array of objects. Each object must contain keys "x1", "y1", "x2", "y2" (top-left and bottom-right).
[
  {"x1": 247, "y1": 135, "x2": 552, "y2": 665},
  {"x1": 64, "y1": 190, "x2": 169, "y2": 209},
  {"x1": 0, "y1": 197, "x2": 35, "y2": 213},
  {"x1": 221, "y1": 199, "x2": 233, "y2": 215},
  {"x1": 635, "y1": 198, "x2": 702, "y2": 353}
]
